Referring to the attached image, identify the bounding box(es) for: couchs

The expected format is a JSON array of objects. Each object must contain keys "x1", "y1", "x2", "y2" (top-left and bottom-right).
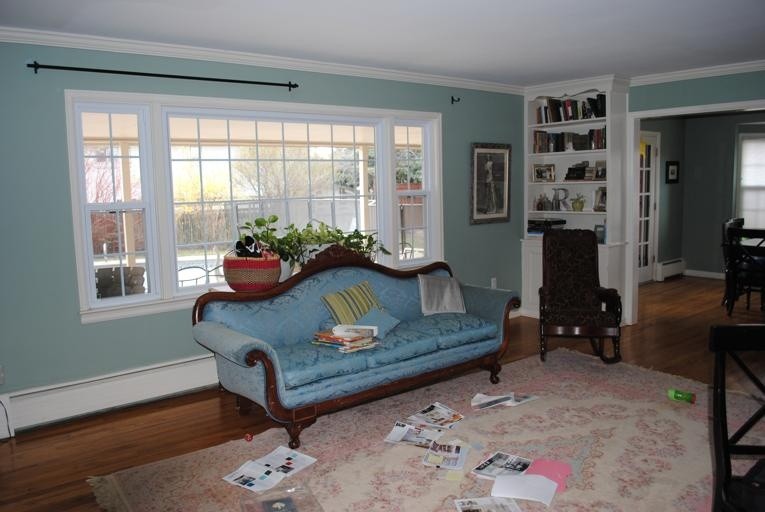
[{"x1": 192, "y1": 242, "x2": 523, "y2": 450}]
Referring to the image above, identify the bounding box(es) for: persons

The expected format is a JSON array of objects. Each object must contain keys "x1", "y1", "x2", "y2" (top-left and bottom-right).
[{"x1": 477, "y1": 152, "x2": 502, "y2": 216}]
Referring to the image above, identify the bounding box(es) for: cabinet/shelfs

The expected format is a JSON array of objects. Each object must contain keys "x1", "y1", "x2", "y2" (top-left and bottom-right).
[{"x1": 520, "y1": 74, "x2": 633, "y2": 329}]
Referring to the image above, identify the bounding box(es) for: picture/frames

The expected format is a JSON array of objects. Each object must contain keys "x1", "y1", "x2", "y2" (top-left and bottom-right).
[
  {"x1": 533, "y1": 164, "x2": 555, "y2": 182},
  {"x1": 470, "y1": 143, "x2": 511, "y2": 225},
  {"x1": 666, "y1": 161, "x2": 680, "y2": 184}
]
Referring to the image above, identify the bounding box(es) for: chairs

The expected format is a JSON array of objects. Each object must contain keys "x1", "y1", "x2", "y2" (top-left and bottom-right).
[
  {"x1": 707, "y1": 324, "x2": 765, "y2": 511},
  {"x1": 720, "y1": 217, "x2": 765, "y2": 317},
  {"x1": 538, "y1": 228, "x2": 622, "y2": 364}
]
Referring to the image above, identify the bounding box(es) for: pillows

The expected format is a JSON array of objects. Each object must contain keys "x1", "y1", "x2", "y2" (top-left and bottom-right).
[
  {"x1": 320, "y1": 280, "x2": 385, "y2": 324},
  {"x1": 356, "y1": 306, "x2": 400, "y2": 340}
]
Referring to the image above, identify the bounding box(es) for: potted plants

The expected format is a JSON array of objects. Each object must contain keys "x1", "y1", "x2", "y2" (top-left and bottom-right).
[{"x1": 222, "y1": 214, "x2": 392, "y2": 294}]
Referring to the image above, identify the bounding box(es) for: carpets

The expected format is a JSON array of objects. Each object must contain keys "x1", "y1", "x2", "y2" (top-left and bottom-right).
[{"x1": 83, "y1": 346, "x2": 764, "y2": 511}]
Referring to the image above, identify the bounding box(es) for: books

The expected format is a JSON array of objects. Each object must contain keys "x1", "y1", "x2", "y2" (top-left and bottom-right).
[
  {"x1": 221, "y1": 444, "x2": 318, "y2": 494},
  {"x1": 527, "y1": 217, "x2": 567, "y2": 235},
  {"x1": 310, "y1": 322, "x2": 381, "y2": 355},
  {"x1": 533, "y1": 93, "x2": 606, "y2": 182}
]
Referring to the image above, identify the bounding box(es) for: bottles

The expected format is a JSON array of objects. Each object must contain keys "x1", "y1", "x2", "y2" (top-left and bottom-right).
[{"x1": 667, "y1": 388, "x2": 697, "y2": 403}]
